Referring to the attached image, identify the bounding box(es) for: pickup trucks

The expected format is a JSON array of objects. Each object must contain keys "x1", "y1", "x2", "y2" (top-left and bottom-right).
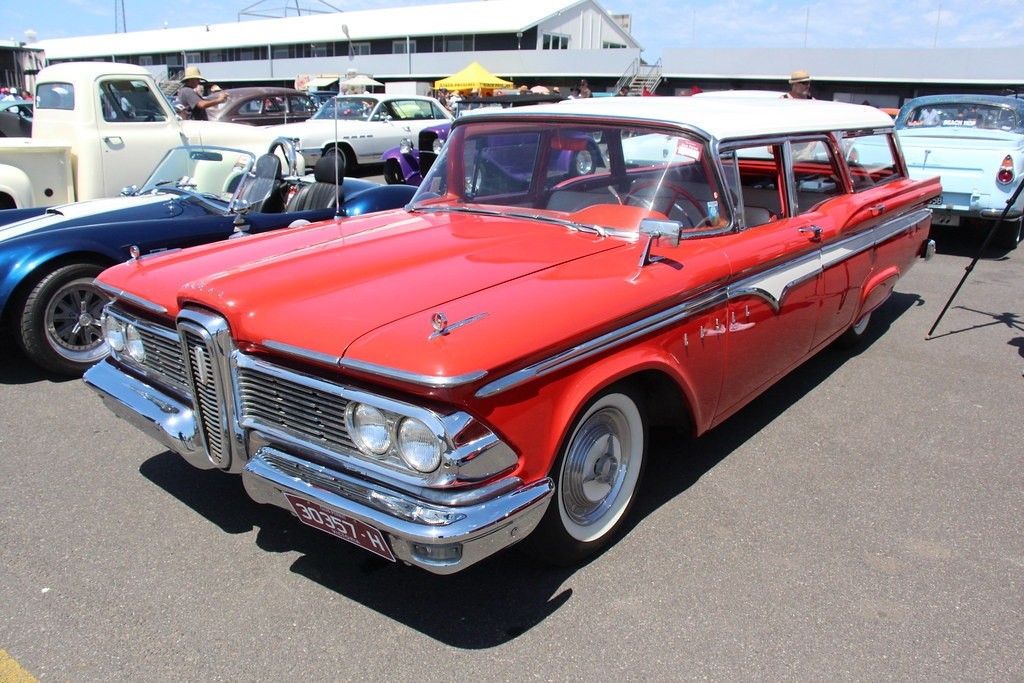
[{"x1": 0, "y1": 60, "x2": 306, "y2": 212}]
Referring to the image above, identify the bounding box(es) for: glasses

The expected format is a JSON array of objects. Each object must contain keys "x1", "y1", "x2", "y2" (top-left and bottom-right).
[{"x1": 798, "y1": 82, "x2": 808, "y2": 86}]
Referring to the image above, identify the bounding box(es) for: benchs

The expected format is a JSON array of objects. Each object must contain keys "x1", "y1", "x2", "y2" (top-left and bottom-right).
[
  {"x1": 632, "y1": 176, "x2": 844, "y2": 220},
  {"x1": 544, "y1": 189, "x2": 771, "y2": 229}
]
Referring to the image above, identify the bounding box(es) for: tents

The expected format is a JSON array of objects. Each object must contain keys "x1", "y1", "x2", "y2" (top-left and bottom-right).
[{"x1": 435, "y1": 62, "x2": 514, "y2": 108}]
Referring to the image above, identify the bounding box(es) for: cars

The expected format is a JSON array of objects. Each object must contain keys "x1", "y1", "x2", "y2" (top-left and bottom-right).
[
  {"x1": 843, "y1": 93, "x2": 1024, "y2": 247},
  {"x1": 256, "y1": 94, "x2": 457, "y2": 176},
  {"x1": 381, "y1": 94, "x2": 607, "y2": 193},
  {"x1": 605, "y1": 90, "x2": 840, "y2": 174},
  {"x1": 0, "y1": 142, "x2": 441, "y2": 382},
  {"x1": 79, "y1": 96, "x2": 943, "y2": 577},
  {"x1": 174, "y1": 86, "x2": 367, "y2": 129}
]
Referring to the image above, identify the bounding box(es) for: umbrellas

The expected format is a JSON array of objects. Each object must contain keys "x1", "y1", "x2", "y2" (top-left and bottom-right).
[
  {"x1": 530, "y1": 86, "x2": 550, "y2": 94},
  {"x1": 340, "y1": 76, "x2": 385, "y2": 94}
]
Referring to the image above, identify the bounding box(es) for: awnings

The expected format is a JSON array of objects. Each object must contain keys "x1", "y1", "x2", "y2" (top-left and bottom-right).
[{"x1": 305, "y1": 78, "x2": 338, "y2": 86}]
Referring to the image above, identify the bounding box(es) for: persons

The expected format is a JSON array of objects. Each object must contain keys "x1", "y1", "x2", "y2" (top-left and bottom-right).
[
  {"x1": 178, "y1": 66, "x2": 229, "y2": 121},
  {"x1": 0, "y1": 87, "x2": 33, "y2": 117},
  {"x1": 553, "y1": 80, "x2": 630, "y2": 100},
  {"x1": 955, "y1": 105, "x2": 983, "y2": 122},
  {"x1": 918, "y1": 108, "x2": 941, "y2": 125},
  {"x1": 767, "y1": 70, "x2": 818, "y2": 160},
  {"x1": 121, "y1": 97, "x2": 132, "y2": 115},
  {"x1": 437, "y1": 86, "x2": 529, "y2": 118},
  {"x1": 361, "y1": 91, "x2": 374, "y2": 116}
]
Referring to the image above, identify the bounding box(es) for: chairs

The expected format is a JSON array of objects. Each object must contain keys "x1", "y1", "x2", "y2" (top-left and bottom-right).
[
  {"x1": 238, "y1": 153, "x2": 284, "y2": 215},
  {"x1": 287, "y1": 153, "x2": 344, "y2": 211}
]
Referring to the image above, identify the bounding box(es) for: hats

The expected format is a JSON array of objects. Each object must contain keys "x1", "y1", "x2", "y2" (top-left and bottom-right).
[
  {"x1": 471, "y1": 89, "x2": 478, "y2": 92},
  {"x1": 570, "y1": 85, "x2": 580, "y2": 93},
  {"x1": 211, "y1": 85, "x2": 221, "y2": 92},
  {"x1": 553, "y1": 87, "x2": 559, "y2": 92},
  {"x1": 180, "y1": 66, "x2": 205, "y2": 83},
  {"x1": 452, "y1": 90, "x2": 459, "y2": 96},
  {"x1": 789, "y1": 71, "x2": 812, "y2": 83}
]
